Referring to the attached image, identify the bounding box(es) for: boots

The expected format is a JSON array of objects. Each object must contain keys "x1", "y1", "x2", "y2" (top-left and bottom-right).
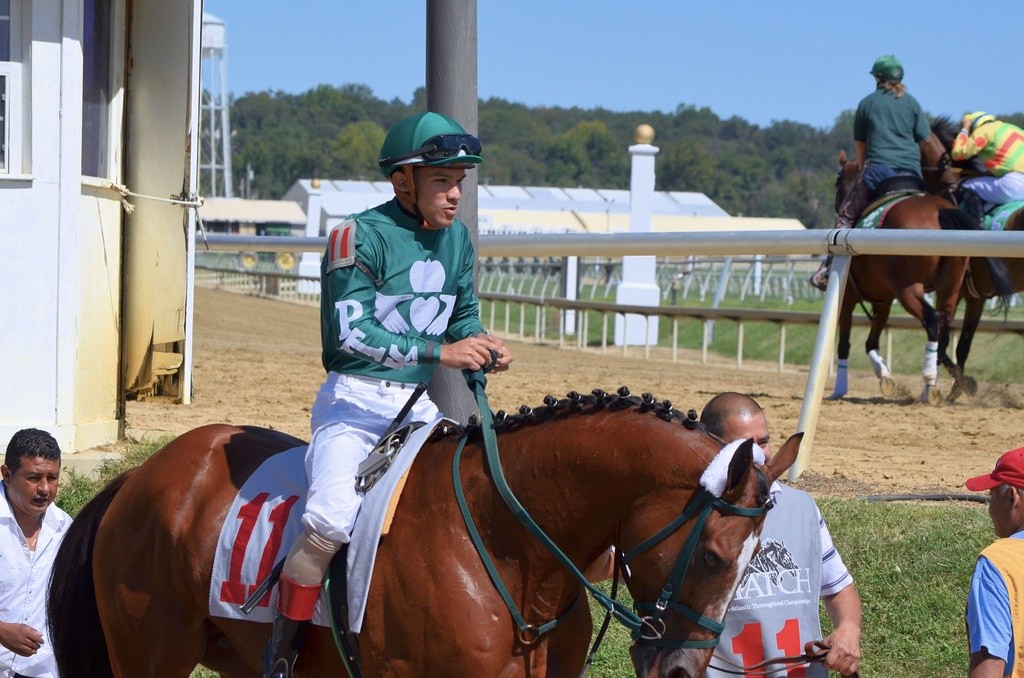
[
  {"x1": 960, "y1": 186, "x2": 984, "y2": 224},
  {"x1": 264, "y1": 571, "x2": 322, "y2": 678},
  {"x1": 811, "y1": 215, "x2": 853, "y2": 285}
]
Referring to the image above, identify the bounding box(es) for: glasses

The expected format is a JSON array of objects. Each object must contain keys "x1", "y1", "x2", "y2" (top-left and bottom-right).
[{"x1": 421, "y1": 133, "x2": 483, "y2": 161}]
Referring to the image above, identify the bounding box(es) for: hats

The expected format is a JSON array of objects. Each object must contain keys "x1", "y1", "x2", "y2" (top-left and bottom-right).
[{"x1": 965, "y1": 447, "x2": 1024, "y2": 492}]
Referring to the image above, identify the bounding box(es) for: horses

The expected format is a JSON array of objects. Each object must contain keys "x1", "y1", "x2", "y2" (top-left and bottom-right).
[
  {"x1": 42, "y1": 386, "x2": 806, "y2": 677},
  {"x1": 820, "y1": 113, "x2": 1024, "y2": 404}
]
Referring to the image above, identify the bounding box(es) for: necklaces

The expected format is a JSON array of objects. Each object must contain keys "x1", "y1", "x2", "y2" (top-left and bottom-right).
[{"x1": 32, "y1": 539, "x2": 37, "y2": 547}]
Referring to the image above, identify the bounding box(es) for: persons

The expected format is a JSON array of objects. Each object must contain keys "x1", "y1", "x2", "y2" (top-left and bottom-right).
[
  {"x1": 824, "y1": 53, "x2": 931, "y2": 277},
  {"x1": 264, "y1": 112, "x2": 514, "y2": 678},
  {"x1": 965, "y1": 446, "x2": 1024, "y2": 678},
  {"x1": 950, "y1": 112, "x2": 1024, "y2": 206},
  {"x1": 585, "y1": 391, "x2": 861, "y2": 678},
  {"x1": 0, "y1": 429, "x2": 74, "y2": 678}
]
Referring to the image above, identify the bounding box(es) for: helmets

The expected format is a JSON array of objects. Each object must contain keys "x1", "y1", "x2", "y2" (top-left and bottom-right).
[
  {"x1": 869, "y1": 55, "x2": 904, "y2": 85},
  {"x1": 378, "y1": 112, "x2": 483, "y2": 179},
  {"x1": 969, "y1": 111, "x2": 995, "y2": 132}
]
